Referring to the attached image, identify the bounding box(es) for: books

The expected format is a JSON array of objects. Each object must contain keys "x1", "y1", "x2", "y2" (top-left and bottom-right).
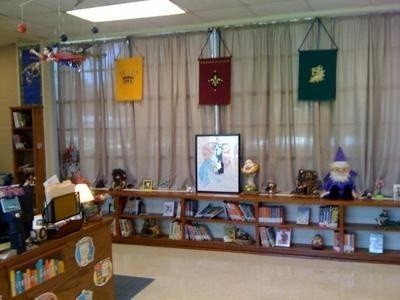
[
  {"x1": 116, "y1": 196, "x2": 385, "y2": 255},
  {"x1": 12, "y1": 110, "x2": 35, "y2": 172}
]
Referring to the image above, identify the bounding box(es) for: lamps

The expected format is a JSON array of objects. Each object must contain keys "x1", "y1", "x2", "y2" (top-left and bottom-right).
[{"x1": 72, "y1": 182, "x2": 95, "y2": 226}]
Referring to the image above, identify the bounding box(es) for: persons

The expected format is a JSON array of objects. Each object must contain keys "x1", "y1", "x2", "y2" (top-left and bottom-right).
[{"x1": 321, "y1": 146, "x2": 357, "y2": 202}]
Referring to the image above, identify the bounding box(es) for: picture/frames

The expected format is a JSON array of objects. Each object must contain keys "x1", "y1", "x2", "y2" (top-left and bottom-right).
[{"x1": 194, "y1": 132, "x2": 242, "y2": 194}]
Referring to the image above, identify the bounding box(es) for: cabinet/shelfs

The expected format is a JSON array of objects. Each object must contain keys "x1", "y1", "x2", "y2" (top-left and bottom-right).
[
  {"x1": 1, "y1": 215, "x2": 116, "y2": 300},
  {"x1": 64, "y1": 182, "x2": 400, "y2": 267},
  {"x1": 8, "y1": 104, "x2": 49, "y2": 216}
]
